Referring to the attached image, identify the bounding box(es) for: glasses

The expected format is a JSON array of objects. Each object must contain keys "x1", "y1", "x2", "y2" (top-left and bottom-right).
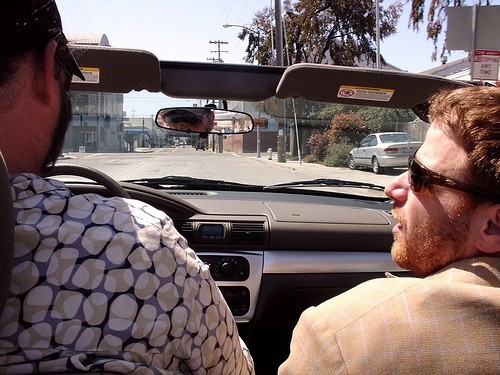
[
  {"x1": 166, "y1": 117, "x2": 200, "y2": 124},
  {"x1": 408, "y1": 146, "x2": 500, "y2": 199}
]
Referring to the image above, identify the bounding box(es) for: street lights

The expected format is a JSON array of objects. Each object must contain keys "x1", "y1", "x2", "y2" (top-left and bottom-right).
[{"x1": 222, "y1": 24, "x2": 261, "y2": 66}]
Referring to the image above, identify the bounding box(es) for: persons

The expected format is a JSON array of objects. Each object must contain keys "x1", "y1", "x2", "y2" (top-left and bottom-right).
[
  {"x1": 275, "y1": 84, "x2": 500, "y2": 375},
  {"x1": 0, "y1": 0, "x2": 256, "y2": 375},
  {"x1": 161, "y1": 107, "x2": 214, "y2": 133}
]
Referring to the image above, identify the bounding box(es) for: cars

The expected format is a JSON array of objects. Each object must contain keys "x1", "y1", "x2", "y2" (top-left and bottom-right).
[{"x1": 347, "y1": 131, "x2": 424, "y2": 175}]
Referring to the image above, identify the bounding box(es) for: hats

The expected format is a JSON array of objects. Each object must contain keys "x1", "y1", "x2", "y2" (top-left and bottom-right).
[
  {"x1": 0, "y1": 0, "x2": 86, "y2": 82},
  {"x1": 162, "y1": 108, "x2": 206, "y2": 117}
]
